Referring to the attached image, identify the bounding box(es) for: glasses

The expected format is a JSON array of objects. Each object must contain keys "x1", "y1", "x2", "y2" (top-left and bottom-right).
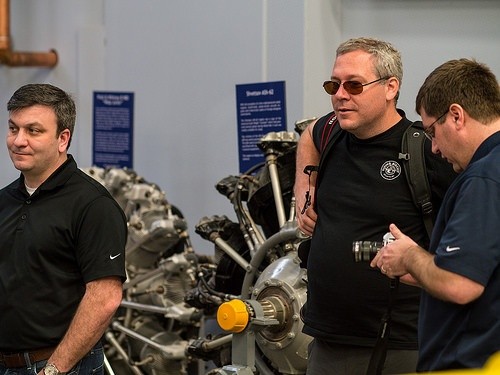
[
  {"x1": 323, "y1": 76, "x2": 390, "y2": 95},
  {"x1": 423, "y1": 108, "x2": 451, "y2": 141}
]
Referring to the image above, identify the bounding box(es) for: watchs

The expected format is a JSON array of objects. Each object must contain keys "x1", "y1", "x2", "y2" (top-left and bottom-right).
[{"x1": 43, "y1": 361, "x2": 69, "y2": 375}]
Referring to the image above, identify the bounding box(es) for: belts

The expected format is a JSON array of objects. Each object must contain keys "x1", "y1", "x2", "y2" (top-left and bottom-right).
[{"x1": 0, "y1": 347, "x2": 55, "y2": 368}]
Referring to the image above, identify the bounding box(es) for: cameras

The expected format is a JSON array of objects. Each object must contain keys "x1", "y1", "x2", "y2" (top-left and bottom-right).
[{"x1": 352, "y1": 232, "x2": 396, "y2": 263}]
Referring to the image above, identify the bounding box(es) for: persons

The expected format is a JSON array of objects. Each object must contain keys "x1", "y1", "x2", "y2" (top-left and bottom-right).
[
  {"x1": 0, "y1": 84, "x2": 130, "y2": 375},
  {"x1": 369, "y1": 58, "x2": 500, "y2": 375},
  {"x1": 293, "y1": 36, "x2": 458, "y2": 375}
]
